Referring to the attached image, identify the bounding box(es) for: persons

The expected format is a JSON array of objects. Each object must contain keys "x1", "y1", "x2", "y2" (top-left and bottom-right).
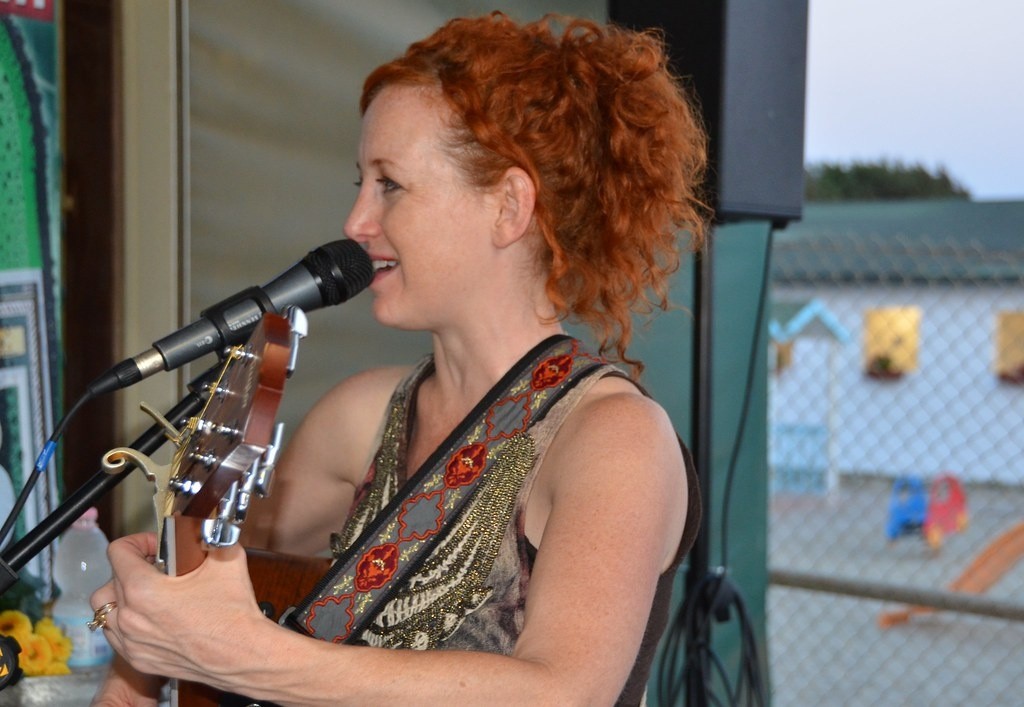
[{"x1": 88, "y1": 10, "x2": 715, "y2": 707}]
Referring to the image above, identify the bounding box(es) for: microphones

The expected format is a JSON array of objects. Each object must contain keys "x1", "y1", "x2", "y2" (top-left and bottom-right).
[{"x1": 88, "y1": 239, "x2": 375, "y2": 396}]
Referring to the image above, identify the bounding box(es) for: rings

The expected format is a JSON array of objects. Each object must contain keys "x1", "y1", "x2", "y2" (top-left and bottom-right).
[{"x1": 86, "y1": 601, "x2": 120, "y2": 632}]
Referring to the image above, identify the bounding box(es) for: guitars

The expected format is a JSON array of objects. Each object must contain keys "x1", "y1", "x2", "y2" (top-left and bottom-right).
[{"x1": 99, "y1": 308, "x2": 313, "y2": 707}]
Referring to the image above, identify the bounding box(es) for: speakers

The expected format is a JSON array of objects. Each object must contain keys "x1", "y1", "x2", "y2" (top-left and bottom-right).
[{"x1": 605, "y1": 1, "x2": 809, "y2": 230}]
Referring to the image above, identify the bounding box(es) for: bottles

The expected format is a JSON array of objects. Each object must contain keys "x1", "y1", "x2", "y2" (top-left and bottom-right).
[{"x1": 50, "y1": 508, "x2": 115, "y2": 666}]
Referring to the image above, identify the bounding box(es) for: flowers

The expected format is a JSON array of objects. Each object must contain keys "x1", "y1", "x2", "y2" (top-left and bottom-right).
[{"x1": 0, "y1": 566, "x2": 71, "y2": 678}]
